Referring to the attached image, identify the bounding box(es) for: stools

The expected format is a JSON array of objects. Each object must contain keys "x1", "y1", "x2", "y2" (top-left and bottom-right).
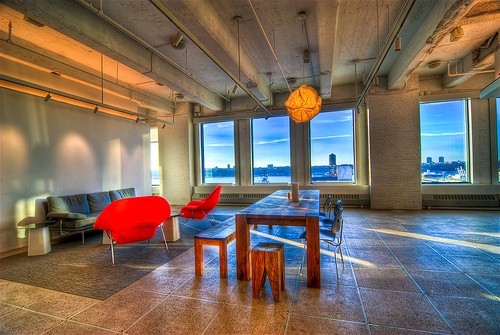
[{"x1": 251, "y1": 242, "x2": 285, "y2": 302}]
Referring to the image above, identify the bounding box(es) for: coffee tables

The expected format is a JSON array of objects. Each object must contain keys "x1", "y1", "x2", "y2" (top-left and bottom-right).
[{"x1": 103, "y1": 213, "x2": 182, "y2": 244}]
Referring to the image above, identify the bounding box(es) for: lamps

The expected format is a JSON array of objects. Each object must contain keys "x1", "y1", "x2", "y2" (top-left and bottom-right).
[
  {"x1": 136, "y1": 118, "x2": 139, "y2": 124},
  {"x1": 44, "y1": 95, "x2": 51, "y2": 102},
  {"x1": 286, "y1": 12, "x2": 322, "y2": 122},
  {"x1": 94, "y1": 107, "x2": 98, "y2": 112},
  {"x1": 232, "y1": 85, "x2": 237, "y2": 93},
  {"x1": 254, "y1": 105, "x2": 259, "y2": 112},
  {"x1": 170, "y1": 32, "x2": 183, "y2": 46},
  {"x1": 374, "y1": 77, "x2": 378, "y2": 86},
  {"x1": 395, "y1": 35, "x2": 401, "y2": 51}
]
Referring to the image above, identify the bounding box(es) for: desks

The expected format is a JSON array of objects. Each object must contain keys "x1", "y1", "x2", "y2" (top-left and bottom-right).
[
  {"x1": 237, "y1": 189, "x2": 320, "y2": 288},
  {"x1": 17, "y1": 221, "x2": 55, "y2": 256}
]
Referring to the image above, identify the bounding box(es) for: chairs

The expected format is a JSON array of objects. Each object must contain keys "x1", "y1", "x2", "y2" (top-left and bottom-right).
[
  {"x1": 94, "y1": 196, "x2": 168, "y2": 264},
  {"x1": 297, "y1": 196, "x2": 343, "y2": 280},
  {"x1": 181, "y1": 186, "x2": 221, "y2": 228}
]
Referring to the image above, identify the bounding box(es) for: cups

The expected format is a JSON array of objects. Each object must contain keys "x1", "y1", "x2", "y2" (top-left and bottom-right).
[
  {"x1": 287, "y1": 192, "x2": 292, "y2": 200},
  {"x1": 291, "y1": 182, "x2": 299, "y2": 202}
]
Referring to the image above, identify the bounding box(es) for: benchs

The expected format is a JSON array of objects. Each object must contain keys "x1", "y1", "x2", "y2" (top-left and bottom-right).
[
  {"x1": 47, "y1": 187, "x2": 136, "y2": 245},
  {"x1": 194, "y1": 215, "x2": 235, "y2": 279}
]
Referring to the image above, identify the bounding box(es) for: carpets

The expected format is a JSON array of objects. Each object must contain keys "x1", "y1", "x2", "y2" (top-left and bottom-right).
[{"x1": 0, "y1": 216, "x2": 232, "y2": 301}]
